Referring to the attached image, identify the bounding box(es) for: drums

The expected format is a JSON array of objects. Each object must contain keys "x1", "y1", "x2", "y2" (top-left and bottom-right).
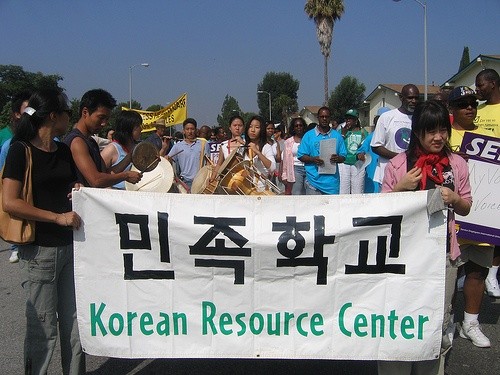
[
  {"x1": 131, "y1": 141, "x2": 160, "y2": 173},
  {"x1": 219, "y1": 159, "x2": 286, "y2": 195},
  {"x1": 191, "y1": 165, "x2": 220, "y2": 195},
  {"x1": 215, "y1": 149, "x2": 243, "y2": 179},
  {"x1": 125, "y1": 156, "x2": 179, "y2": 193}
]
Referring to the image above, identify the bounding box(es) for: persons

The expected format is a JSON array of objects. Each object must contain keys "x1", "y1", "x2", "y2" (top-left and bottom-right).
[
  {"x1": 445, "y1": 86, "x2": 492, "y2": 348},
  {"x1": 65, "y1": 88, "x2": 143, "y2": 189},
  {"x1": 2, "y1": 88, "x2": 86, "y2": 375},
  {"x1": 377, "y1": 99, "x2": 472, "y2": 375},
  {"x1": 457, "y1": 69, "x2": 500, "y2": 297},
  {"x1": 371, "y1": 83, "x2": 421, "y2": 191},
  {"x1": 0, "y1": 92, "x2": 382, "y2": 264}
]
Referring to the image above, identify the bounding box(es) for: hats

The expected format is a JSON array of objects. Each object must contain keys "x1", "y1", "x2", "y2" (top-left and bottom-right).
[
  {"x1": 344, "y1": 108, "x2": 359, "y2": 119},
  {"x1": 374, "y1": 106, "x2": 392, "y2": 116},
  {"x1": 152, "y1": 119, "x2": 167, "y2": 127},
  {"x1": 448, "y1": 85, "x2": 477, "y2": 102}
]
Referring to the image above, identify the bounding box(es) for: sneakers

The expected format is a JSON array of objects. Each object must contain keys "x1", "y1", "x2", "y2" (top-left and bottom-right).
[
  {"x1": 459, "y1": 320, "x2": 491, "y2": 348},
  {"x1": 9, "y1": 251, "x2": 19, "y2": 263},
  {"x1": 484, "y1": 277, "x2": 500, "y2": 297}
]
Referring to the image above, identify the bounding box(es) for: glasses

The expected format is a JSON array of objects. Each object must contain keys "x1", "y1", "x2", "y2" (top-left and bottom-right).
[
  {"x1": 455, "y1": 101, "x2": 478, "y2": 109},
  {"x1": 63, "y1": 108, "x2": 72, "y2": 117}
]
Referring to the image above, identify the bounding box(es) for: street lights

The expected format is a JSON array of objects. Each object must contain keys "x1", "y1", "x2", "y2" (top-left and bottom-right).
[
  {"x1": 257, "y1": 91, "x2": 271, "y2": 122},
  {"x1": 129, "y1": 63, "x2": 149, "y2": 109},
  {"x1": 393, "y1": 0, "x2": 427, "y2": 103}
]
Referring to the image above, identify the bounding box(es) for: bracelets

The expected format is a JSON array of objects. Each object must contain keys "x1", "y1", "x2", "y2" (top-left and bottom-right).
[{"x1": 62, "y1": 213, "x2": 67, "y2": 226}]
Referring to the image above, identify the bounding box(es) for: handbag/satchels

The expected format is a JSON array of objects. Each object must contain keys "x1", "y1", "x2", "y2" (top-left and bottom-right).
[{"x1": 0, "y1": 141, "x2": 35, "y2": 244}]
